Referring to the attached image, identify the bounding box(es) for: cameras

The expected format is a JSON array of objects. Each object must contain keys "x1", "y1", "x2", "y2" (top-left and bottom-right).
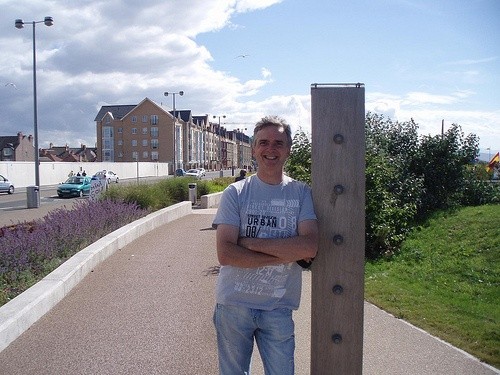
[{"x1": 295, "y1": 258, "x2": 316, "y2": 268}]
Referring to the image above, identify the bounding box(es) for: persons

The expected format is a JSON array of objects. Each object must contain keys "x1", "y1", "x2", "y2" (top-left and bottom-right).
[
  {"x1": 77, "y1": 172, "x2": 81, "y2": 176},
  {"x1": 212, "y1": 115, "x2": 321, "y2": 375},
  {"x1": 68, "y1": 170, "x2": 75, "y2": 177},
  {"x1": 234, "y1": 170, "x2": 247, "y2": 182},
  {"x1": 82, "y1": 171, "x2": 86, "y2": 176}
]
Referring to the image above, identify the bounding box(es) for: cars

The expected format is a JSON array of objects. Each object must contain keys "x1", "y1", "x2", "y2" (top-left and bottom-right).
[
  {"x1": 93, "y1": 170, "x2": 119, "y2": 184},
  {"x1": 0, "y1": 175, "x2": 15, "y2": 195},
  {"x1": 197, "y1": 168, "x2": 206, "y2": 178},
  {"x1": 186, "y1": 169, "x2": 202, "y2": 180},
  {"x1": 56, "y1": 176, "x2": 91, "y2": 198}
]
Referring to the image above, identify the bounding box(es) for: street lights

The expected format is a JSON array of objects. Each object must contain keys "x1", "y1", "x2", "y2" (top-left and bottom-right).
[
  {"x1": 164, "y1": 90, "x2": 184, "y2": 177},
  {"x1": 213, "y1": 115, "x2": 226, "y2": 170},
  {"x1": 237, "y1": 127, "x2": 247, "y2": 169},
  {"x1": 14, "y1": 16, "x2": 54, "y2": 186}
]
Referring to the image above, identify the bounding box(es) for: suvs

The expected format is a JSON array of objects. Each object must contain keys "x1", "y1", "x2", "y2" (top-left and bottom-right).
[{"x1": 175, "y1": 168, "x2": 185, "y2": 177}]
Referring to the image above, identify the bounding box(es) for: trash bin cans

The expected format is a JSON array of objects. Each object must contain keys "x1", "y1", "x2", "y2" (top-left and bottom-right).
[
  {"x1": 26, "y1": 185, "x2": 39, "y2": 209},
  {"x1": 187, "y1": 183, "x2": 197, "y2": 205}
]
